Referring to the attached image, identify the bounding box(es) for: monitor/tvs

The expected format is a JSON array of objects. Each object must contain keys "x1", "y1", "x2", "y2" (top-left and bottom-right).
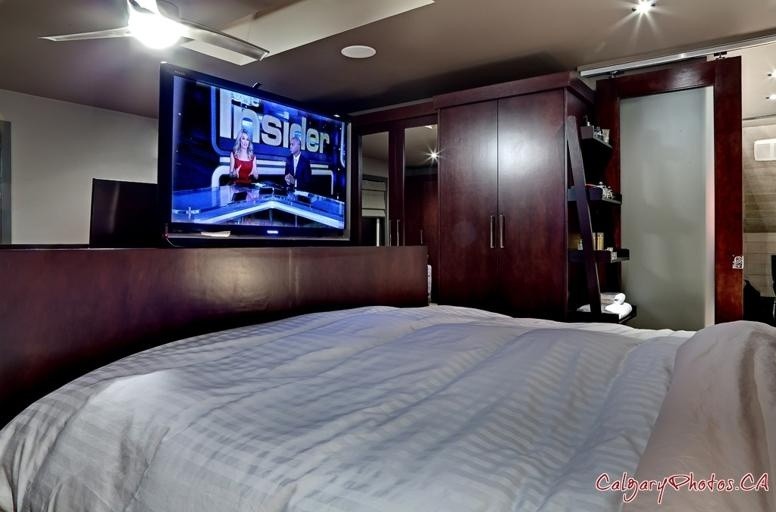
[{"x1": 152, "y1": 62, "x2": 363, "y2": 246}]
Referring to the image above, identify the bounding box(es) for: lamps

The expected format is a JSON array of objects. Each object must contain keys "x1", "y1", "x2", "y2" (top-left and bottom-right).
[{"x1": 35, "y1": 0, "x2": 272, "y2": 68}]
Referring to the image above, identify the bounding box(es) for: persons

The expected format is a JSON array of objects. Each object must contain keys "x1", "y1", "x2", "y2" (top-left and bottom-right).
[
  {"x1": 230, "y1": 128, "x2": 258, "y2": 180},
  {"x1": 285, "y1": 137, "x2": 312, "y2": 189}
]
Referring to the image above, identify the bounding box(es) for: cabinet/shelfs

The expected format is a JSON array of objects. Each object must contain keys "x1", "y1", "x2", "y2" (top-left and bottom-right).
[{"x1": 428, "y1": 73, "x2": 640, "y2": 323}]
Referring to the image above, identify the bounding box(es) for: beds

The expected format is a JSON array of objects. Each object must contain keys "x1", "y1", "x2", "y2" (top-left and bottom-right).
[{"x1": 0, "y1": 243, "x2": 776, "y2": 512}]
